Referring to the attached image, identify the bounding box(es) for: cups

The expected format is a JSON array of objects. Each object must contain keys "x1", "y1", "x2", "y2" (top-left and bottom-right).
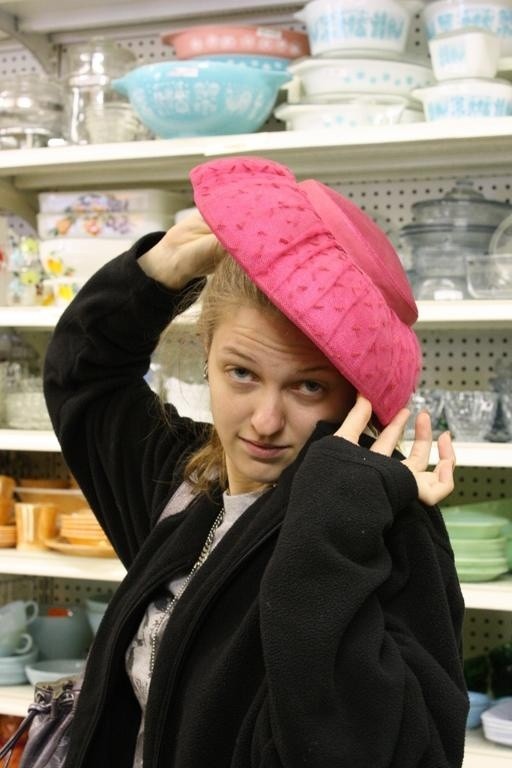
[
  {"x1": 1, "y1": 599, "x2": 39, "y2": 654},
  {"x1": 401, "y1": 390, "x2": 498, "y2": 441}
]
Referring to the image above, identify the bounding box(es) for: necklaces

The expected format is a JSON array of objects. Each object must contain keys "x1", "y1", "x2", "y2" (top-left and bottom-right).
[{"x1": 148, "y1": 505, "x2": 227, "y2": 676}]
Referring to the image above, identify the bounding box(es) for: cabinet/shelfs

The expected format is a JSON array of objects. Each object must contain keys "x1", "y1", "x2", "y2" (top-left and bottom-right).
[{"x1": 0, "y1": 1, "x2": 512, "y2": 768}]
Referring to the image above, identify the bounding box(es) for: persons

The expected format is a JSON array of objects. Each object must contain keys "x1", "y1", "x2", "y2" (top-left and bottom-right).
[{"x1": 43, "y1": 156, "x2": 472, "y2": 766}]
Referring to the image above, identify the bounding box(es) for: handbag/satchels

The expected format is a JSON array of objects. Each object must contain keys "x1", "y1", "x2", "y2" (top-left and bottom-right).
[{"x1": 0, "y1": 677, "x2": 84, "y2": 768}]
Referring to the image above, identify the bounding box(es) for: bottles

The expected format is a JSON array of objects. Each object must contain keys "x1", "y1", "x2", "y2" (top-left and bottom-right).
[{"x1": 64, "y1": 37, "x2": 136, "y2": 143}]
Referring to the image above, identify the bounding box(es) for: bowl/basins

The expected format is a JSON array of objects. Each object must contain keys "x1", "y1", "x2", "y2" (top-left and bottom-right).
[
  {"x1": 37, "y1": 190, "x2": 182, "y2": 310},
  {"x1": 23, "y1": 606, "x2": 90, "y2": 692},
  {"x1": 110, "y1": 1, "x2": 508, "y2": 136},
  {"x1": 465, "y1": 253, "x2": 512, "y2": 298},
  {"x1": 84, "y1": 592, "x2": 114, "y2": 634},
  {"x1": 466, "y1": 690, "x2": 488, "y2": 730}
]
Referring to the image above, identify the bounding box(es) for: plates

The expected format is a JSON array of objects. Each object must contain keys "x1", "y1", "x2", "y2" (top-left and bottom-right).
[
  {"x1": 440, "y1": 498, "x2": 510, "y2": 585},
  {"x1": 480, "y1": 697, "x2": 512, "y2": 744},
  {"x1": 1, "y1": 476, "x2": 118, "y2": 557}
]
